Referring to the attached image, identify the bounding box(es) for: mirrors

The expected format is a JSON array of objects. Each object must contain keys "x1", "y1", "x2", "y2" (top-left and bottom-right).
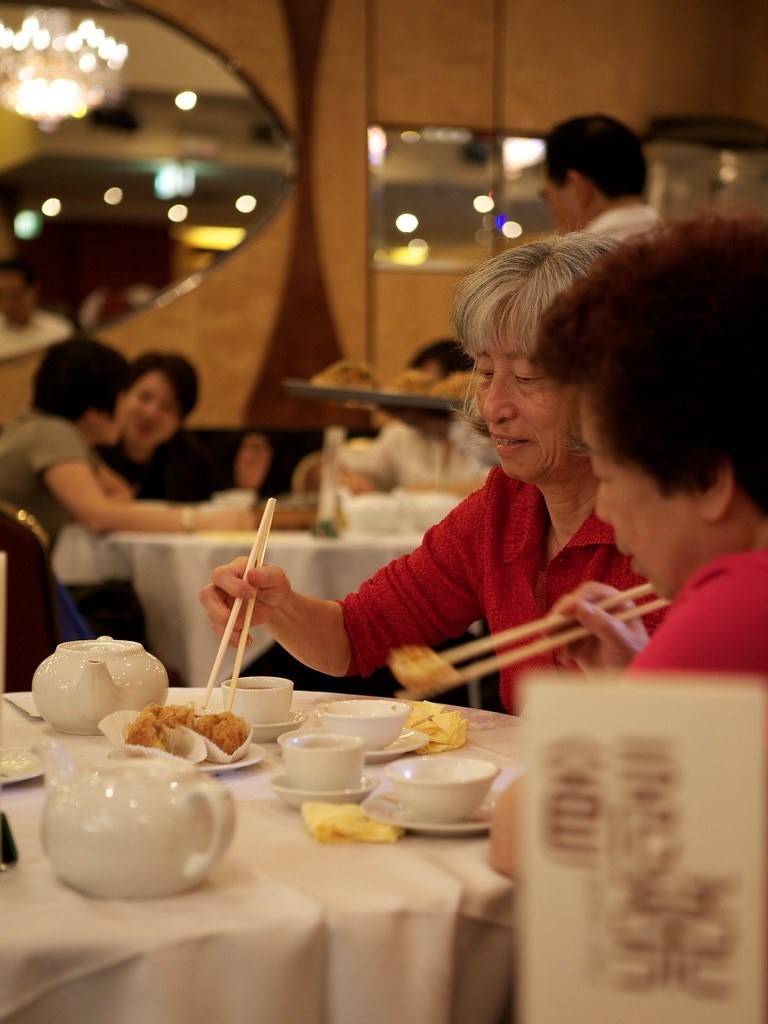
[{"x1": 1, "y1": 0, "x2": 304, "y2": 368}]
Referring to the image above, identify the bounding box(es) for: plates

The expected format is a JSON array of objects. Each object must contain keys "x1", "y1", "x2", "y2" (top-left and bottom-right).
[
  {"x1": 360, "y1": 767, "x2": 524, "y2": 834},
  {"x1": 191, "y1": 741, "x2": 265, "y2": 772},
  {"x1": 300, "y1": 714, "x2": 430, "y2": 764},
  {"x1": 0, "y1": 770, "x2": 45, "y2": 785},
  {"x1": 269, "y1": 773, "x2": 377, "y2": 811},
  {"x1": 211, "y1": 711, "x2": 308, "y2": 742}
]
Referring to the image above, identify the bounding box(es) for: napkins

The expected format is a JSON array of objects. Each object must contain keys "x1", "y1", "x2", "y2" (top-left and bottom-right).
[
  {"x1": 300, "y1": 802, "x2": 405, "y2": 844},
  {"x1": 406, "y1": 699, "x2": 470, "y2": 756}
]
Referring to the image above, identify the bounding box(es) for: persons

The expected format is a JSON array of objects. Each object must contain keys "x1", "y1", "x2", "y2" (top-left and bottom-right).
[
  {"x1": 0, "y1": 338, "x2": 255, "y2": 538},
  {"x1": 259, "y1": 339, "x2": 489, "y2": 503},
  {"x1": 0, "y1": 260, "x2": 74, "y2": 360},
  {"x1": 97, "y1": 351, "x2": 271, "y2": 501},
  {"x1": 537, "y1": 112, "x2": 663, "y2": 236},
  {"x1": 199, "y1": 233, "x2": 674, "y2": 716},
  {"x1": 490, "y1": 217, "x2": 768, "y2": 874}
]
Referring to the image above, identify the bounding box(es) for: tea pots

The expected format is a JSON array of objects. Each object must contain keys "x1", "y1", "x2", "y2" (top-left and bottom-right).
[
  {"x1": 27, "y1": 739, "x2": 232, "y2": 897},
  {"x1": 335, "y1": 483, "x2": 398, "y2": 532},
  {"x1": 32, "y1": 636, "x2": 168, "y2": 736}
]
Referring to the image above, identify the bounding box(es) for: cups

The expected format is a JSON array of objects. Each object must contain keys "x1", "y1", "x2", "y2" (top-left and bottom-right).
[
  {"x1": 220, "y1": 675, "x2": 293, "y2": 724},
  {"x1": 277, "y1": 729, "x2": 363, "y2": 788},
  {"x1": 383, "y1": 754, "x2": 499, "y2": 822}
]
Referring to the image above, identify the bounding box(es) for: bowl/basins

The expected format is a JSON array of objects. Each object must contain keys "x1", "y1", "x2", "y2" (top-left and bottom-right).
[{"x1": 316, "y1": 699, "x2": 413, "y2": 751}]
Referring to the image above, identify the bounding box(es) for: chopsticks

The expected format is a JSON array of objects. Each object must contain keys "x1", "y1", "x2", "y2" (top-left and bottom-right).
[
  {"x1": 395, "y1": 581, "x2": 673, "y2": 702},
  {"x1": 201, "y1": 497, "x2": 276, "y2": 713}
]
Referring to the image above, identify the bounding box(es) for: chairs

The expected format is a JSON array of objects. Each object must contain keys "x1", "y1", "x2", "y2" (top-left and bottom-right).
[{"x1": 0, "y1": 502, "x2": 63, "y2": 694}]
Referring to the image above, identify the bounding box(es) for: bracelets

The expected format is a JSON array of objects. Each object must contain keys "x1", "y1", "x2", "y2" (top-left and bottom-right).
[{"x1": 182, "y1": 507, "x2": 191, "y2": 530}]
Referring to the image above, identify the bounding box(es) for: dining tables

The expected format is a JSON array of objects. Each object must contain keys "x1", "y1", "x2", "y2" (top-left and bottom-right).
[
  {"x1": 0, "y1": 687, "x2": 521, "y2": 1024},
  {"x1": 51, "y1": 493, "x2": 472, "y2": 689}
]
